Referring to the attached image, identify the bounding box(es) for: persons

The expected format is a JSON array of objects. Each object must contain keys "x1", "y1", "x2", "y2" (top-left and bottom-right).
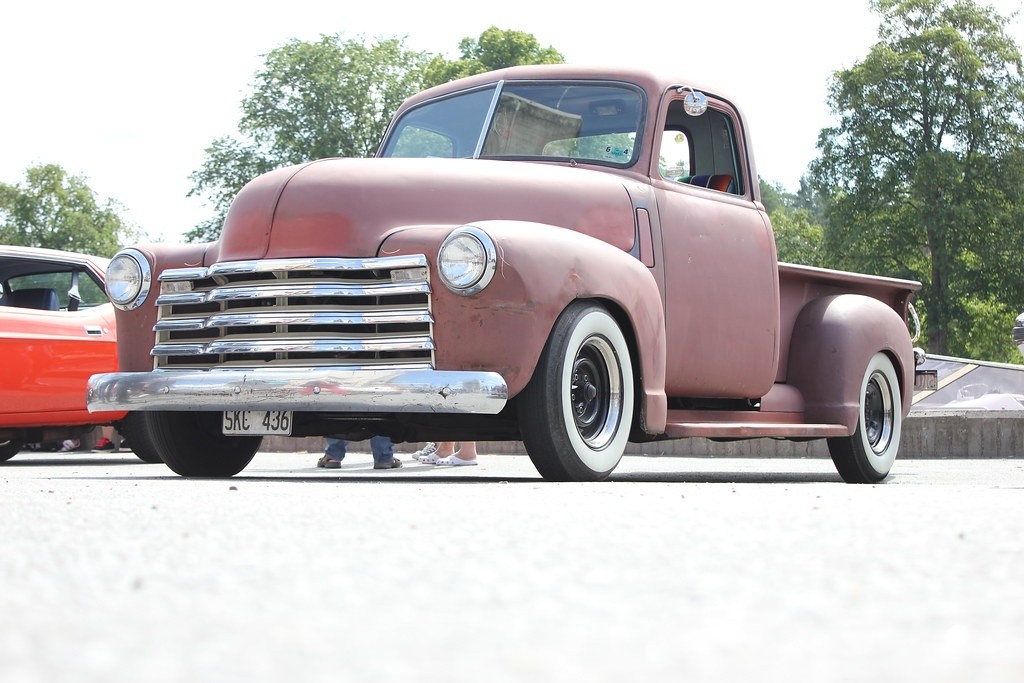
[
  {"x1": 412, "y1": 442, "x2": 440, "y2": 461},
  {"x1": 318, "y1": 436, "x2": 404, "y2": 471},
  {"x1": 419, "y1": 442, "x2": 479, "y2": 468},
  {"x1": 91, "y1": 425, "x2": 132, "y2": 453}
]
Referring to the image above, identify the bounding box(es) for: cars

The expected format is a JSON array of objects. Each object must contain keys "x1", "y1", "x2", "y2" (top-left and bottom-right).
[{"x1": 0, "y1": 242, "x2": 162, "y2": 465}]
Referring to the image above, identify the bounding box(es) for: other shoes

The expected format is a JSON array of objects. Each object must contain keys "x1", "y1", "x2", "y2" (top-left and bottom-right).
[{"x1": 57, "y1": 437, "x2": 81, "y2": 452}]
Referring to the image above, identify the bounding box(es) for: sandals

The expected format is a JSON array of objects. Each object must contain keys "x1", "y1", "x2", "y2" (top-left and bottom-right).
[
  {"x1": 374, "y1": 457, "x2": 402, "y2": 469},
  {"x1": 317, "y1": 453, "x2": 342, "y2": 469}
]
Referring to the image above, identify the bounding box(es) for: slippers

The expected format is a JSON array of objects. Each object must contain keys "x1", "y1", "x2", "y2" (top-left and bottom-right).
[
  {"x1": 436, "y1": 455, "x2": 478, "y2": 466},
  {"x1": 418, "y1": 452, "x2": 441, "y2": 463}
]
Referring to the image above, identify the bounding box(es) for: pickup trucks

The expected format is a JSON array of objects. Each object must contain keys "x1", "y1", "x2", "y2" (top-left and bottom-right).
[{"x1": 88, "y1": 62, "x2": 939, "y2": 485}]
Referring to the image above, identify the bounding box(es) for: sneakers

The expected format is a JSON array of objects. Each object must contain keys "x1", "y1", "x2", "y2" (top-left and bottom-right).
[
  {"x1": 412, "y1": 442, "x2": 436, "y2": 459},
  {"x1": 91, "y1": 436, "x2": 115, "y2": 453},
  {"x1": 119, "y1": 438, "x2": 132, "y2": 452}
]
